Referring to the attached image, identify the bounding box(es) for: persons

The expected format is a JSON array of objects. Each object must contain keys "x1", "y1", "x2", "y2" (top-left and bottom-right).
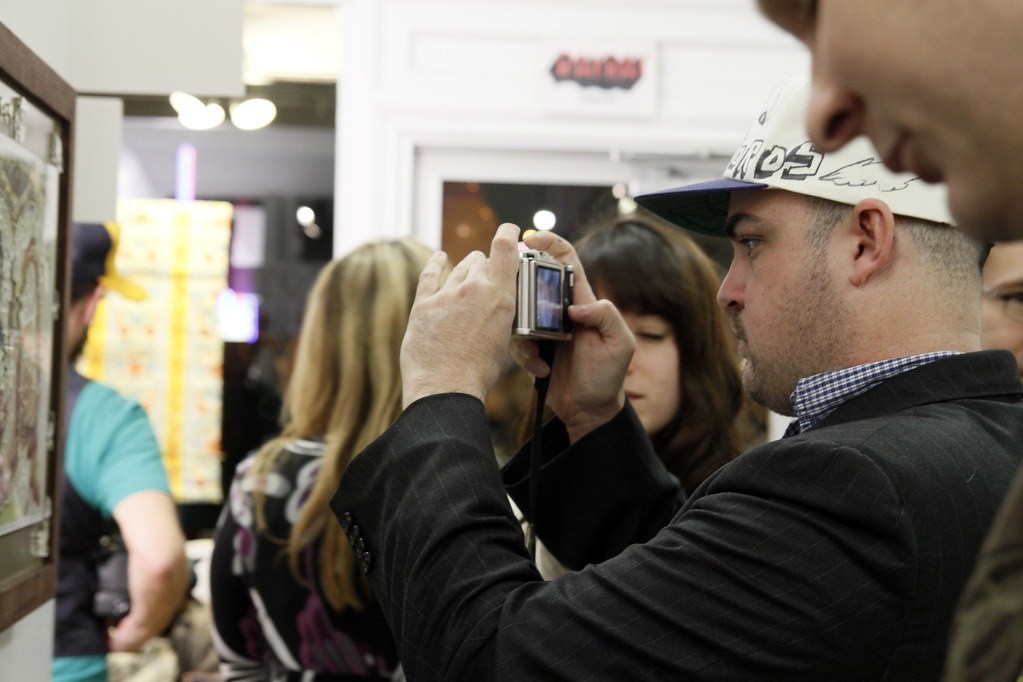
[
  {"x1": 54, "y1": 222, "x2": 188, "y2": 682},
  {"x1": 328, "y1": 0, "x2": 1022, "y2": 681},
  {"x1": 210, "y1": 240, "x2": 443, "y2": 681}
]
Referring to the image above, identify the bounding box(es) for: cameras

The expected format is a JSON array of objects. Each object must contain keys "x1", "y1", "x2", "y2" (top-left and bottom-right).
[{"x1": 509, "y1": 248, "x2": 576, "y2": 341}]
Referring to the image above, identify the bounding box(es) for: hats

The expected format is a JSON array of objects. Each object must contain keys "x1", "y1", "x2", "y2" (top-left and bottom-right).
[
  {"x1": 69, "y1": 223, "x2": 111, "y2": 279},
  {"x1": 634, "y1": 85, "x2": 958, "y2": 235}
]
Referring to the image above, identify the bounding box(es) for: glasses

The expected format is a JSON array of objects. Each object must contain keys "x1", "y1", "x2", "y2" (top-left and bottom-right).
[{"x1": 980, "y1": 291, "x2": 1023, "y2": 322}]
